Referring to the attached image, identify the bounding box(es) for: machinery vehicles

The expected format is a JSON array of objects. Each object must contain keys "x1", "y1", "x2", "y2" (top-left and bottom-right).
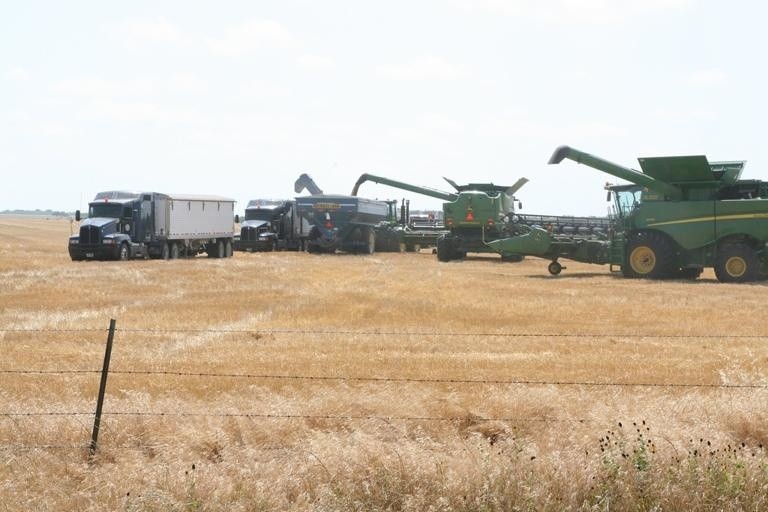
[
  {"x1": 356, "y1": 173, "x2": 578, "y2": 273},
  {"x1": 548, "y1": 144, "x2": 768, "y2": 282}
]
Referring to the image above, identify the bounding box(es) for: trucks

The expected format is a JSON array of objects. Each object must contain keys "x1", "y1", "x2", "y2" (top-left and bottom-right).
[
  {"x1": 294, "y1": 196, "x2": 389, "y2": 256},
  {"x1": 69, "y1": 191, "x2": 236, "y2": 261},
  {"x1": 233, "y1": 200, "x2": 315, "y2": 252}
]
